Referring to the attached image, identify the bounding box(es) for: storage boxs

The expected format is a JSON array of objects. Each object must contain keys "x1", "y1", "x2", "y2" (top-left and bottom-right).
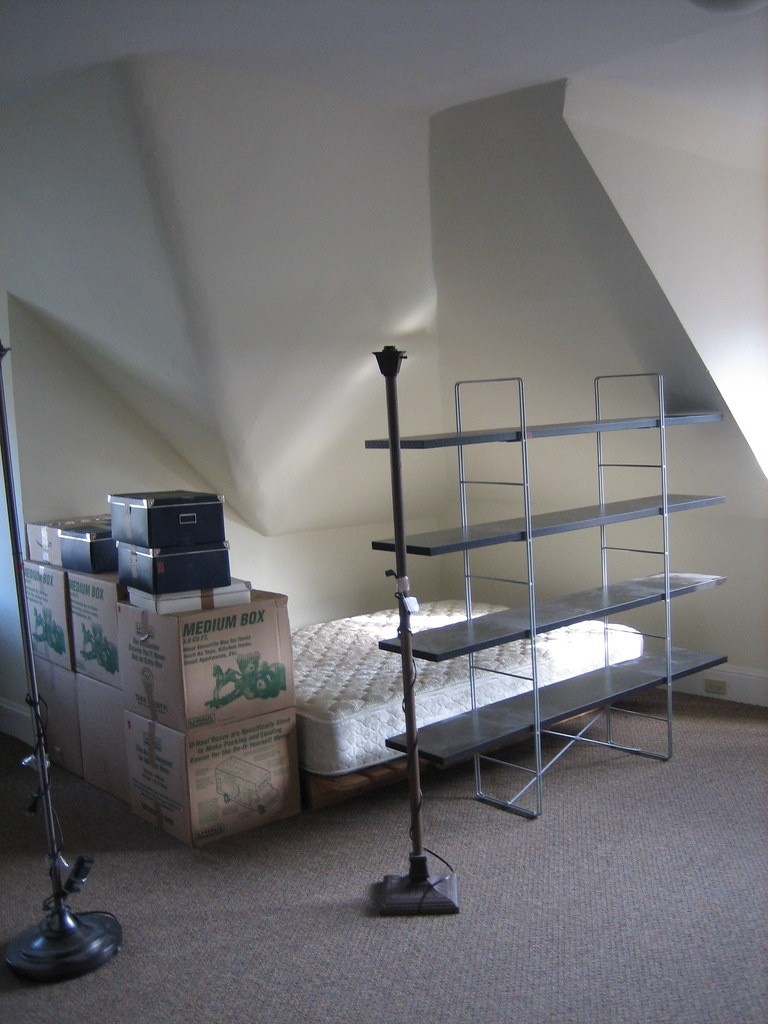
[{"x1": 22, "y1": 488, "x2": 303, "y2": 850}]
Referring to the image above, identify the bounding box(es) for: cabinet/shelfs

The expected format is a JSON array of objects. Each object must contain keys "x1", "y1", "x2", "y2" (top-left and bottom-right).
[{"x1": 364, "y1": 372, "x2": 726, "y2": 822}]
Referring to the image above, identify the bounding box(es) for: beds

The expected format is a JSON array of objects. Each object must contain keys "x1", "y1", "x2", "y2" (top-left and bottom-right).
[{"x1": 290, "y1": 599, "x2": 644, "y2": 811}]
